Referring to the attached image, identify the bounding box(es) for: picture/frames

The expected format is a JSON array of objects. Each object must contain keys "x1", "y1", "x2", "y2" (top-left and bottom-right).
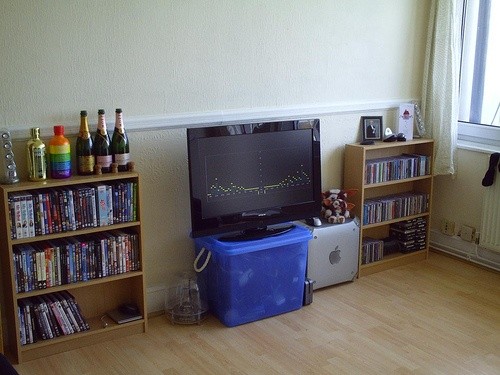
[{"x1": 362, "y1": 116, "x2": 382, "y2": 142}]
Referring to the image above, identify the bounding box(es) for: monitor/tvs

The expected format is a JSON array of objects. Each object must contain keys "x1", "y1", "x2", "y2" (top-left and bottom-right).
[{"x1": 185, "y1": 118, "x2": 322, "y2": 244}]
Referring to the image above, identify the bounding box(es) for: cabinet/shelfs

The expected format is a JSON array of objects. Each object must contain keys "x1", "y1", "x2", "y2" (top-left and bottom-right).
[
  {"x1": 0, "y1": 170, "x2": 147, "y2": 364},
  {"x1": 344, "y1": 139, "x2": 434, "y2": 279}
]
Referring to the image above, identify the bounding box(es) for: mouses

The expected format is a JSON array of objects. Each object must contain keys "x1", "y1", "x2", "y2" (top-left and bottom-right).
[{"x1": 304, "y1": 217, "x2": 321, "y2": 226}]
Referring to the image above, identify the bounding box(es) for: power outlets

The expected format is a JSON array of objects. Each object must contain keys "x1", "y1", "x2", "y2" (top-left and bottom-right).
[
  {"x1": 442, "y1": 221, "x2": 453, "y2": 236},
  {"x1": 461, "y1": 225, "x2": 473, "y2": 242}
]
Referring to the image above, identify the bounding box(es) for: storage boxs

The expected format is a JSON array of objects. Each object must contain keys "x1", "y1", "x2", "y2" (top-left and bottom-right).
[{"x1": 190, "y1": 221, "x2": 313, "y2": 327}]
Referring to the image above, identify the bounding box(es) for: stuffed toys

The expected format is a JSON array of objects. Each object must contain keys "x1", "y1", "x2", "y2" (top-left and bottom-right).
[{"x1": 321, "y1": 188, "x2": 358, "y2": 223}]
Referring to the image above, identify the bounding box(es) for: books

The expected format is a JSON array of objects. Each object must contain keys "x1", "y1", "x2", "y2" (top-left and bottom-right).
[
  {"x1": 12, "y1": 227, "x2": 140, "y2": 294},
  {"x1": 106, "y1": 308, "x2": 143, "y2": 324},
  {"x1": 17, "y1": 290, "x2": 90, "y2": 345},
  {"x1": 362, "y1": 236, "x2": 384, "y2": 264},
  {"x1": 7, "y1": 181, "x2": 137, "y2": 240},
  {"x1": 365, "y1": 153, "x2": 432, "y2": 184},
  {"x1": 363, "y1": 190, "x2": 430, "y2": 225}
]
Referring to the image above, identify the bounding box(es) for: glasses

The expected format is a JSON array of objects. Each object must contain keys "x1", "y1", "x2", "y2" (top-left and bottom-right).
[{"x1": 384, "y1": 133, "x2": 406, "y2": 142}]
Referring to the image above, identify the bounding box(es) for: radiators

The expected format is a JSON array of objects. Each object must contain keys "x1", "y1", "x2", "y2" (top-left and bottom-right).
[{"x1": 479, "y1": 154, "x2": 500, "y2": 253}]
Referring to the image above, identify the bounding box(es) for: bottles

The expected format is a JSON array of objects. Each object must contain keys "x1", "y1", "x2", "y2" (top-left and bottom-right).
[
  {"x1": 111, "y1": 108, "x2": 133, "y2": 172},
  {"x1": 25, "y1": 127, "x2": 48, "y2": 182},
  {"x1": 76, "y1": 110, "x2": 96, "y2": 176},
  {"x1": 48, "y1": 125, "x2": 72, "y2": 179},
  {"x1": 93, "y1": 109, "x2": 113, "y2": 174}
]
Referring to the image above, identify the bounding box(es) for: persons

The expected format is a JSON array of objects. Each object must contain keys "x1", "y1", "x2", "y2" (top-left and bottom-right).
[{"x1": 367, "y1": 120, "x2": 376, "y2": 138}]
